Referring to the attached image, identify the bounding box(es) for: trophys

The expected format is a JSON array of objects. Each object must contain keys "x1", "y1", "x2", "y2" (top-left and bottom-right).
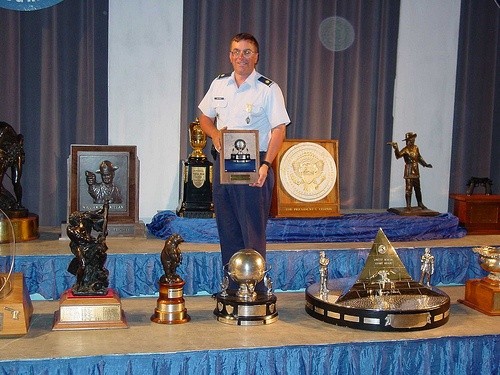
[
  {"x1": 176, "y1": 117, "x2": 217, "y2": 157},
  {"x1": 151, "y1": 234, "x2": 191, "y2": 324},
  {"x1": 210, "y1": 249, "x2": 280, "y2": 326},
  {"x1": 472, "y1": 244, "x2": 500, "y2": 287}
]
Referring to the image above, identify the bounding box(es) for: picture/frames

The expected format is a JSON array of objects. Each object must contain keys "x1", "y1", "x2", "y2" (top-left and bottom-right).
[
  {"x1": 220, "y1": 129, "x2": 260, "y2": 184},
  {"x1": 70, "y1": 143, "x2": 137, "y2": 224}
]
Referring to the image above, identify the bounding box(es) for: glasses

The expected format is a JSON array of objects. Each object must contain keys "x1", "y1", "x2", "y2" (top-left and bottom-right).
[{"x1": 231, "y1": 47, "x2": 256, "y2": 57}]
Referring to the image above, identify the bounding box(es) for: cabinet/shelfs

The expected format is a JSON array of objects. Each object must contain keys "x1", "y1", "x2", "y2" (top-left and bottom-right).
[{"x1": 449, "y1": 193, "x2": 500, "y2": 235}]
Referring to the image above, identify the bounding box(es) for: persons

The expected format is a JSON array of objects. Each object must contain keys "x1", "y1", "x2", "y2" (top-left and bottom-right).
[
  {"x1": 11, "y1": 134, "x2": 26, "y2": 207},
  {"x1": 319, "y1": 250, "x2": 330, "y2": 296},
  {"x1": 386, "y1": 132, "x2": 433, "y2": 209},
  {"x1": 419, "y1": 247, "x2": 435, "y2": 287},
  {"x1": 67, "y1": 204, "x2": 109, "y2": 291},
  {"x1": 85, "y1": 161, "x2": 122, "y2": 204},
  {"x1": 197, "y1": 33, "x2": 291, "y2": 285}
]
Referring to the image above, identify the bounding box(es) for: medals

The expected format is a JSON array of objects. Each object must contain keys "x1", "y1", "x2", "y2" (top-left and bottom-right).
[{"x1": 51, "y1": 200, "x2": 129, "y2": 331}]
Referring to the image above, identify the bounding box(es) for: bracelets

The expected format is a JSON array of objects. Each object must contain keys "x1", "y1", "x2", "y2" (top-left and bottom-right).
[{"x1": 261, "y1": 161, "x2": 271, "y2": 167}]
